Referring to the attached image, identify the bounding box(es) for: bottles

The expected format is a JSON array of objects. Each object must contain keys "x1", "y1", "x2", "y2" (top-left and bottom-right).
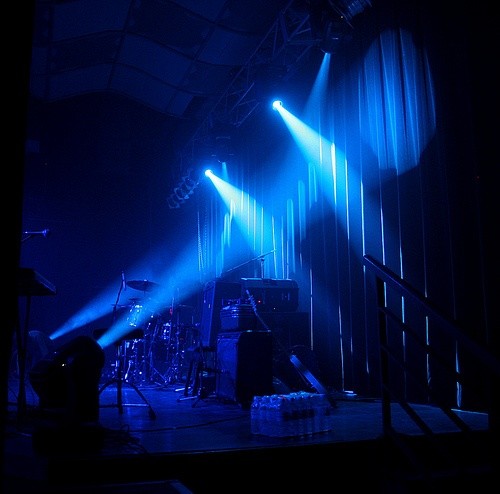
[{"x1": 250, "y1": 392, "x2": 332, "y2": 438}]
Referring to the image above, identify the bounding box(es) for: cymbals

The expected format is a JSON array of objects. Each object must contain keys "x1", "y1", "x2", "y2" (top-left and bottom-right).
[
  {"x1": 127, "y1": 280, "x2": 164, "y2": 291},
  {"x1": 94, "y1": 328, "x2": 143, "y2": 339},
  {"x1": 110, "y1": 303, "x2": 133, "y2": 308},
  {"x1": 129, "y1": 298, "x2": 160, "y2": 303}
]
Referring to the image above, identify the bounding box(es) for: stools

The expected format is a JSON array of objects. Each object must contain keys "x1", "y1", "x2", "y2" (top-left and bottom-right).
[{"x1": 93, "y1": 328, "x2": 156, "y2": 420}]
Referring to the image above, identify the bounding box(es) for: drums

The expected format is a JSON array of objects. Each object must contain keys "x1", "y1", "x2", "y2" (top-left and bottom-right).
[
  {"x1": 127, "y1": 305, "x2": 156, "y2": 332},
  {"x1": 159, "y1": 343, "x2": 189, "y2": 358},
  {"x1": 160, "y1": 322, "x2": 199, "y2": 340},
  {"x1": 126, "y1": 342, "x2": 150, "y2": 359},
  {"x1": 170, "y1": 279, "x2": 197, "y2": 308}
]
